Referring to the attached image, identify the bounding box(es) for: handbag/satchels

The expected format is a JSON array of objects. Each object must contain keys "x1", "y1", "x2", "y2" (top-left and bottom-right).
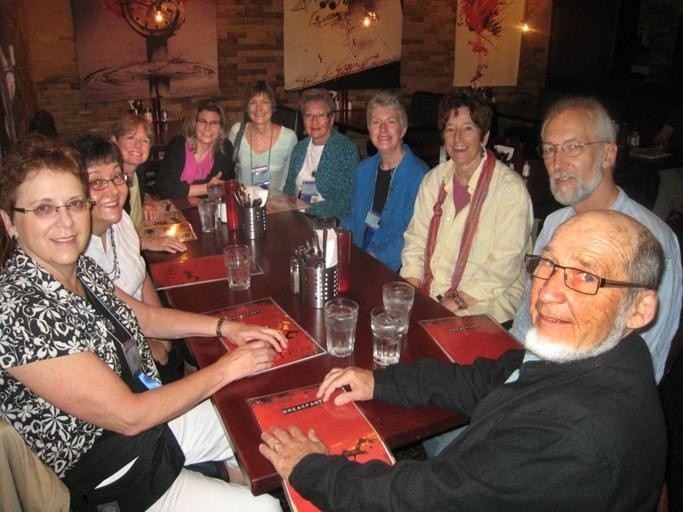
[{"x1": 62, "y1": 386, "x2": 186, "y2": 507}]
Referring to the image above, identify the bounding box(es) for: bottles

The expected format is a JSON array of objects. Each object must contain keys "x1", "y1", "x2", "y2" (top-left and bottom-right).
[
  {"x1": 162, "y1": 109, "x2": 168, "y2": 123},
  {"x1": 152, "y1": 98, "x2": 161, "y2": 122},
  {"x1": 334, "y1": 227, "x2": 352, "y2": 292},
  {"x1": 290, "y1": 258, "x2": 299, "y2": 295},
  {"x1": 217, "y1": 197, "x2": 226, "y2": 223},
  {"x1": 144, "y1": 108, "x2": 153, "y2": 122},
  {"x1": 334, "y1": 110, "x2": 353, "y2": 123},
  {"x1": 151, "y1": 122, "x2": 169, "y2": 144},
  {"x1": 335, "y1": 88, "x2": 353, "y2": 112},
  {"x1": 223, "y1": 180, "x2": 240, "y2": 230}
]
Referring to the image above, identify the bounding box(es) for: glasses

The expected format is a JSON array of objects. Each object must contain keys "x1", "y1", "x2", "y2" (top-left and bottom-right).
[
  {"x1": 89, "y1": 169, "x2": 128, "y2": 192},
  {"x1": 535, "y1": 138, "x2": 610, "y2": 161},
  {"x1": 301, "y1": 110, "x2": 329, "y2": 121},
  {"x1": 197, "y1": 119, "x2": 221, "y2": 128},
  {"x1": 13, "y1": 198, "x2": 97, "y2": 218},
  {"x1": 524, "y1": 252, "x2": 654, "y2": 297}
]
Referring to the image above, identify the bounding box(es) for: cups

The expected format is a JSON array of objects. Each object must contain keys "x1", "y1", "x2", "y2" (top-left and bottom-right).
[
  {"x1": 370, "y1": 303, "x2": 409, "y2": 370},
  {"x1": 381, "y1": 282, "x2": 414, "y2": 314},
  {"x1": 206, "y1": 183, "x2": 224, "y2": 201},
  {"x1": 223, "y1": 243, "x2": 253, "y2": 292},
  {"x1": 324, "y1": 297, "x2": 360, "y2": 358},
  {"x1": 198, "y1": 199, "x2": 219, "y2": 233},
  {"x1": 314, "y1": 215, "x2": 337, "y2": 249}
]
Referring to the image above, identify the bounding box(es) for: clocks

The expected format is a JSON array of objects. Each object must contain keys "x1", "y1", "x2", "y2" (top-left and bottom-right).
[{"x1": 121, "y1": 0, "x2": 184, "y2": 39}]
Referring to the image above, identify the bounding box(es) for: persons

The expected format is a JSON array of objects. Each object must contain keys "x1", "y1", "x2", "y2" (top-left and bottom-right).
[
  {"x1": 401, "y1": 87, "x2": 533, "y2": 330},
  {"x1": 2, "y1": 135, "x2": 288, "y2": 512},
  {"x1": 63, "y1": 115, "x2": 188, "y2": 378},
  {"x1": 509, "y1": 93, "x2": 681, "y2": 383},
  {"x1": 155, "y1": 78, "x2": 298, "y2": 197},
  {"x1": 258, "y1": 210, "x2": 665, "y2": 512},
  {"x1": 283, "y1": 88, "x2": 428, "y2": 273}
]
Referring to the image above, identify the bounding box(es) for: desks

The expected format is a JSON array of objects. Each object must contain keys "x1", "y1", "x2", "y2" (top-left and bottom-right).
[
  {"x1": 129, "y1": 184, "x2": 524, "y2": 498},
  {"x1": 624, "y1": 146, "x2": 682, "y2": 223}
]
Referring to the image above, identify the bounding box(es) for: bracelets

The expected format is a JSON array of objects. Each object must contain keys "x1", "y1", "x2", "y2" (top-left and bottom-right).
[
  {"x1": 216, "y1": 318, "x2": 225, "y2": 337},
  {"x1": 453, "y1": 295, "x2": 468, "y2": 309}
]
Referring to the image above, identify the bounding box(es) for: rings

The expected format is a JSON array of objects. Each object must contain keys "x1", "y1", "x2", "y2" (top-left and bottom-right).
[{"x1": 273, "y1": 442, "x2": 281, "y2": 452}]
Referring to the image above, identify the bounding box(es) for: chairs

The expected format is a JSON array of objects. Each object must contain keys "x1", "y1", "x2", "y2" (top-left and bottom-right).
[
  {"x1": 0, "y1": 417, "x2": 227, "y2": 512},
  {"x1": 267, "y1": 88, "x2": 647, "y2": 214}
]
